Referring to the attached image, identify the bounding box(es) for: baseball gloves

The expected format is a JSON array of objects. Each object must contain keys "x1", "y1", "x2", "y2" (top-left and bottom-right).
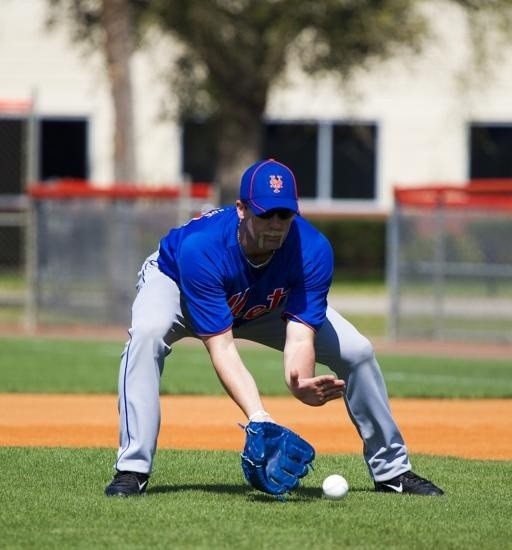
[{"x1": 242, "y1": 419, "x2": 315, "y2": 496}]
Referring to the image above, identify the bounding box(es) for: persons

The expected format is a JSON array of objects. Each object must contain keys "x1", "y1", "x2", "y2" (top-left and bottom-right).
[{"x1": 103, "y1": 157, "x2": 446, "y2": 498}]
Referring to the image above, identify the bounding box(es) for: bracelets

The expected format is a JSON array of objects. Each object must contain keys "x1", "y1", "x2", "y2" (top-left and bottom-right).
[{"x1": 247, "y1": 410, "x2": 269, "y2": 421}]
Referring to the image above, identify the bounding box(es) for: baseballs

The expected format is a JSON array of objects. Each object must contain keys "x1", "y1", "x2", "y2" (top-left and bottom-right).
[{"x1": 322, "y1": 475, "x2": 347, "y2": 498}]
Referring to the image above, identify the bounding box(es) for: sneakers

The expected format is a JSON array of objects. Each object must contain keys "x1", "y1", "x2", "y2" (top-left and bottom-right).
[
  {"x1": 104, "y1": 469, "x2": 149, "y2": 496},
  {"x1": 375, "y1": 470, "x2": 444, "y2": 497}
]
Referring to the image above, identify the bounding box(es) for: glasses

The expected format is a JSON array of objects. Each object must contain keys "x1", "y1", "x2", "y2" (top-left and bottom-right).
[{"x1": 255, "y1": 206, "x2": 296, "y2": 220}]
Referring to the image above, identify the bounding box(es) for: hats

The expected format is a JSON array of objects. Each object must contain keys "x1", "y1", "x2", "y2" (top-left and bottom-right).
[{"x1": 240, "y1": 159, "x2": 300, "y2": 218}]
